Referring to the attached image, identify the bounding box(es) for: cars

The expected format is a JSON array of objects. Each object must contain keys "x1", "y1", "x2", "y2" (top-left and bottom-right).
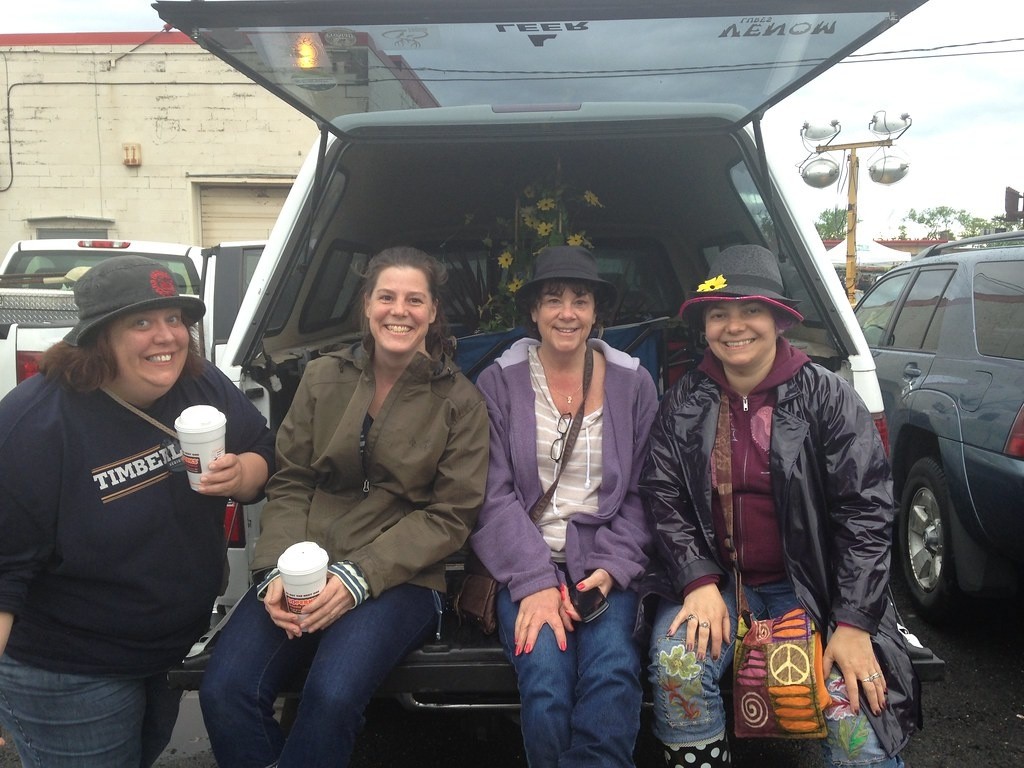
[{"x1": 1, "y1": 238, "x2": 215, "y2": 401}]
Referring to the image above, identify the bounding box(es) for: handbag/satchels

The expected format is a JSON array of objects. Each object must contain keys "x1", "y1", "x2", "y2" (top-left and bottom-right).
[
  {"x1": 733, "y1": 607, "x2": 833, "y2": 739},
  {"x1": 445, "y1": 572, "x2": 501, "y2": 636}
]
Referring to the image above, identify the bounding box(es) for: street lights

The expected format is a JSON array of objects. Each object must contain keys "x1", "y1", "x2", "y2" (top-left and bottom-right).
[{"x1": 796, "y1": 110, "x2": 912, "y2": 312}]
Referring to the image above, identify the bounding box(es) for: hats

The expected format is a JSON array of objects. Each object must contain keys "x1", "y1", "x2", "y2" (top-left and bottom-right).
[
  {"x1": 514, "y1": 244, "x2": 617, "y2": 308},
  {"x1": 685, "y1": 244, "x2": 804, "y2": 309},
  {"x1": 681, "y1": 292, "x2": 803, "y2": 335},
  {"x1": 63, "y1": 254, "x2": 208, "y2": 348}
]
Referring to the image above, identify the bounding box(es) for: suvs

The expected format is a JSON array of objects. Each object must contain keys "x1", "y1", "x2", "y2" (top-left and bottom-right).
[
  {"x1": 149, "y1": 0, "x2": 925, "y2": 715},
  {"x1": 853, "y1": 231, "x2": 1024, "y2": 629}
]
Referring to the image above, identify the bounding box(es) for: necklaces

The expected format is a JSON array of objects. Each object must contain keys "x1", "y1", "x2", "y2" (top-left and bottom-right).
[{"x1": 546, "y1": 371, "x2": 583, "y2": 403}]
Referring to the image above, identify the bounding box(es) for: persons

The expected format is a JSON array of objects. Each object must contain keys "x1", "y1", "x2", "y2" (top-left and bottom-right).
[
  {"x1": 0, "y1": 256, "x2": 275, "y2": 768},
  {"x1": 199, "y1": 246, "x2": 489, "y2": 768},
  {"x1": 468, "y1": 247, "x2": 660, "y2": 768},
  {"x1": 639, "y1": 244, "x2": 917, "y2": 768}
]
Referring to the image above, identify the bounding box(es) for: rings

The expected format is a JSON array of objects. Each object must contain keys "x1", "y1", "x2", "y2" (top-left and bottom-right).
[
  {"x1": 862, "y1": 677, "x2": 873, "y2": 683},
  {"x1": 871, "y1": 672, "x2": 879, "y2": 679},
  {"x1": 687, "y1": 614, "x2": 696, "y2": 622},
  {"x1": 699, "y1": 622, "x2": 710, "y2": 628}
]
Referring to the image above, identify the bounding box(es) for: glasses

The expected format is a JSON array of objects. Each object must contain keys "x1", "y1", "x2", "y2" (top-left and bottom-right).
[{"x1": 550, "y1": 412, "x2": 572, "y2": 463}]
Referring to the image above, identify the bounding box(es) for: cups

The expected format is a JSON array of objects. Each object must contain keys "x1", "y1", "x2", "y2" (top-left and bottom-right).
[
  {"x1": 276, "y1": 540, "x2": 329, "y2": 632},
  {"x1": 174, "y1": 403, "x2": 228, "y2": 492}
]
